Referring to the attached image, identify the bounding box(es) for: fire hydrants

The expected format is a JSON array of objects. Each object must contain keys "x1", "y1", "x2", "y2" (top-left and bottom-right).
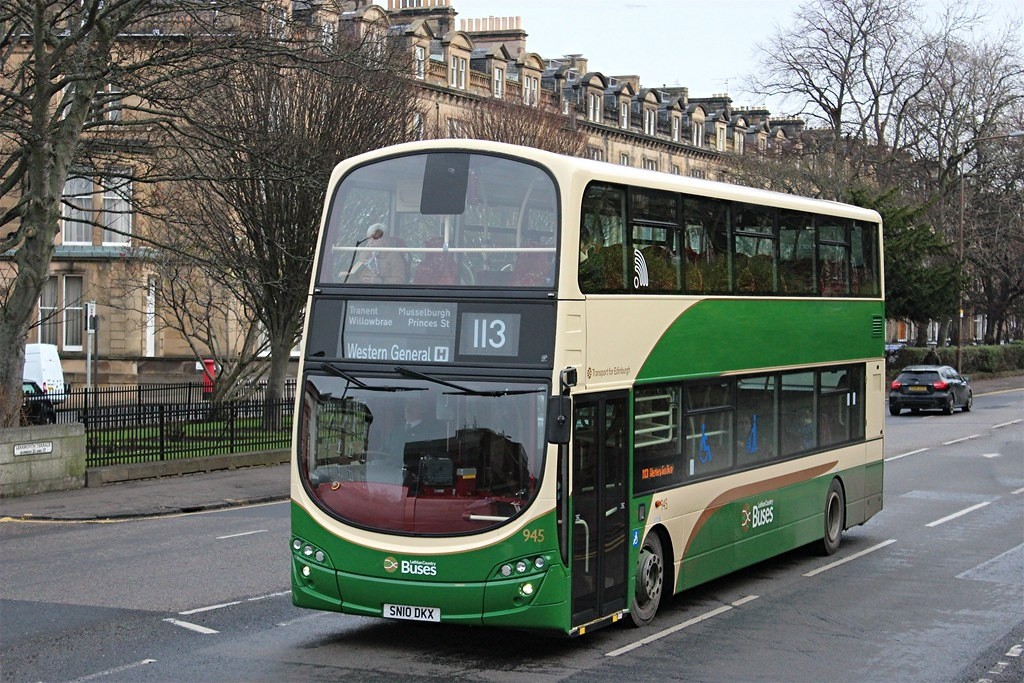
[{"x1": 202, "y1": 359, "x2": 215, "y2": 397}]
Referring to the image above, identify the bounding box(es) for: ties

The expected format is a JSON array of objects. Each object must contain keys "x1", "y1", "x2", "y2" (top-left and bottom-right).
[{"x1": 405, "y1": 423, "x2": 411, "y2": 431}]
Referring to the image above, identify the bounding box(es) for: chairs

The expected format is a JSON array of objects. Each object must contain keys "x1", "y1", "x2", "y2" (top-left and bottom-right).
[
  {"x1": 579, "y1": 420, "x2": 861, "y2": 481},
  {"x1": 351, "y1": 243, "x2": 877, "y2": 295}
]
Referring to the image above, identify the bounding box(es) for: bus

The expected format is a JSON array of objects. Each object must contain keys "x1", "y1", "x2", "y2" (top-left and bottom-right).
[{"x1": 289, "y1": 138, "x2": 886, "y2": 638}]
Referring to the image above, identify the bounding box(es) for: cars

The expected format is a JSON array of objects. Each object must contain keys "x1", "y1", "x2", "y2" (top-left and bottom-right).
[{"x1": 885, "y1": 342, "x2": 907, "y2": 354}]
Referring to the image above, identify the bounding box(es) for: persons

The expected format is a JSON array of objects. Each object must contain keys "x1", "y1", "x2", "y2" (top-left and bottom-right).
[
  {"x1": 349, "y1": 223, "x2": 753, "y2": 294},
  {"x1": 734, "y1": 415, "x2": 757, "y2": 468},
  {"x1": 371, "y1": 396, "x2": 428, "y2": 471},
  {"x1": 924, "y1": 347, "x2": 941, "y2": 367}
]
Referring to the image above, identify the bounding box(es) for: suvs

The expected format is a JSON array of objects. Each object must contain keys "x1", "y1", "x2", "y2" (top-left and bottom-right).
[
  {"x1": 23, "y1": 380, "x2": 56, "y2": 425},
  {"x1": 889, "y1": 365, "x2": 974, "y2": 416}
]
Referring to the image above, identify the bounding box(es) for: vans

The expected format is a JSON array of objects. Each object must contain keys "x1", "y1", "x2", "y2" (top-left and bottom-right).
[{"x1": 23, "y1": 343, "x2": 65, "y2": 404}]
